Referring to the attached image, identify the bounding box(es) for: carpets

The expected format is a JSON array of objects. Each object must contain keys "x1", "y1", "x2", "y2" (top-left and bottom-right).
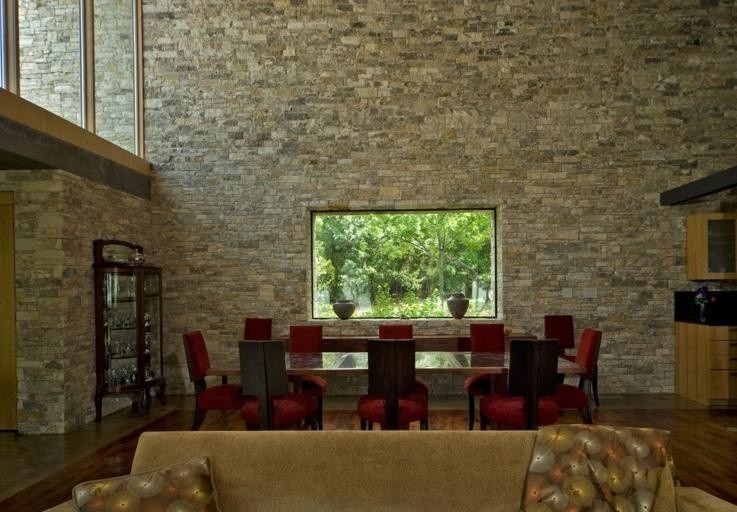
[{"x1": 0, "y1": 406, "x2": 737, "y2": 511}]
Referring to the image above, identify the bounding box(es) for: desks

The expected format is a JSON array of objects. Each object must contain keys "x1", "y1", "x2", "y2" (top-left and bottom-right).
[
  {"x1": 207, "y1": 352, "x2": 587, "y2": 426},
  {"x1": 278, "y1": 335, "x2": 539, "y2": 351}
]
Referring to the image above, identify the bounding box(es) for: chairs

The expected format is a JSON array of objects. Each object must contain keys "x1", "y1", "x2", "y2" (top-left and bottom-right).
[
  {"x1": 182, "y1": 330, "x2": 247, "y2": 430},
  {"x1": 284, "y1": 325, "x2": 327, "y2": 429},
  {"x1": 545, "y1": 314, "x2": 601, "y2": 408},
  {"x1": 358, "y1": 339, "x2": 428, "y2": 430},
  {"x1": 378, "y1": 325, "x2": 413, "y2": 344},
  {"x1": 553, "y1": 330, "x2": 603, "y2": 431},
  {"x1": 237, "y1": 340, "x2": 316, "y2": 428},
  {"x1": 242, "y1": 317, "x2": 273, "y2": 340},
  {"x1": 461, "y1": 323, "x2": 508, "y2": 430},
  {"x1": 477, "y1": 343, "x2": 565, "y2": 430}
]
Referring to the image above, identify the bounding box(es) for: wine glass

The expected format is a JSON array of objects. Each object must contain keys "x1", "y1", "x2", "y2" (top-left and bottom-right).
[{"x1": 104, "y1": 271, "x2": 158, "y2": 386}]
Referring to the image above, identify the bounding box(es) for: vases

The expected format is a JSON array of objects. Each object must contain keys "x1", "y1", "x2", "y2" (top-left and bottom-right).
[
  {"x1": 447, "y1": 292, "x2": 470, "y2": 320},
  {"x1": 332, "y1": 299, "x2": 356, "y2": 321}
]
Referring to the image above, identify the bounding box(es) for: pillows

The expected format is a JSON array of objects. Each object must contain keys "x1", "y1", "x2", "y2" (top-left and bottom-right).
[
  {"x1": 522, "y1": 420, "x2": 674, "y2": 512},
  {"x1": 67, "y1": 457, "x2": 217, "y2": 511}
]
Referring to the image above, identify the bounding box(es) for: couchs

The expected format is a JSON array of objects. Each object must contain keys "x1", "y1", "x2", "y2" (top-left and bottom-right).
[{"x1": 40, "y1": 425, "x2": 737, "y2": 512}]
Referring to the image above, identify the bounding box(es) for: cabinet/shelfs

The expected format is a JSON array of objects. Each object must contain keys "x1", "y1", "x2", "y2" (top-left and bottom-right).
[
  {"x1": 685, "y1": 211, "x2": 737, "y2": 283},
  {"x1": 674, "y1": 317, "x2": 737, "y2": 410},
  {"x1": 92, "y1": 235, "x2": 166, "y2": 422}
]
